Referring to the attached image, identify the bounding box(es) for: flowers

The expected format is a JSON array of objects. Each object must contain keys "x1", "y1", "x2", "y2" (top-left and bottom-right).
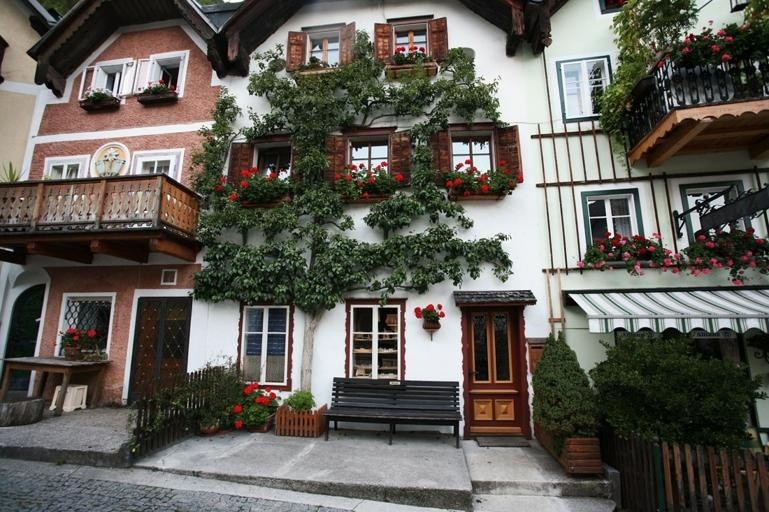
[
  {"x1": 572, "y1": 229, "x2": 682, "y2": 279},
  {"x1": 394, "y1": 46, "x2": 429, "y2": 64},
  {"x1": 447, "y1": 159, "x2": 520, "y2": 198},
  {"x1": 218, "y1": 166, "x2": 277, "y2": 201},
  {"x1": 413, "y1": 300, "x2": 445, "y2": 322},
  {"x1": 682, "y1": 224, "x2": 768, "y2": 289},
  {"x1": 334, "y1": 162, "x2": 405, "y2": 198},
  {"x1": 136, "y1": 80, "x2": 176, "y2": 96},
  {"x1": 231, "y1": 382, "x2": 277, "y2": 432},
  {"x1": 58, "y1": 325, "x2": 98, "y2": 352}
]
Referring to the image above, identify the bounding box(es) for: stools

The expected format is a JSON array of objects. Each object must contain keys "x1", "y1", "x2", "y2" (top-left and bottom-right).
[{"x1": 49, "y1": 382, "x2": 89, "y2": 415}]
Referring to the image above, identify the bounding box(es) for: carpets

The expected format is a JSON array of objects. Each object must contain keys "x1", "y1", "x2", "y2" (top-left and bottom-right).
[{"x1": 473, "y1": 434, "x2": 531, "y2": 449}]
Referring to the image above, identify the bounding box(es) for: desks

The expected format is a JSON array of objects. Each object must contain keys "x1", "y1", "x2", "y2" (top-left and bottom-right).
[{"x1": 0, "y1": 354, "x2": 113, "y2": 416}]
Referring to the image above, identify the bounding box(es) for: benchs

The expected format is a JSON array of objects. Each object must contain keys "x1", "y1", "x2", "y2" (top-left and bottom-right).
[{"x1": 321, "y1": 375, "x2": 467, "y2": 448}]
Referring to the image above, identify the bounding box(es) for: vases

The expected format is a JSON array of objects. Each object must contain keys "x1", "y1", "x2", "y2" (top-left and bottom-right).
[
  {"x1": 422, "y1": 320, "x2": 442, "y2": 334},
  {"x1": 136, "y1": 92, "x2": 177, "y2": 105},
  {"x1": 248, "y1": 413, "x2": 275, "y2": 433},
  {"x1": 63, "y1": 345, "x2": 80, "y2": 360}
]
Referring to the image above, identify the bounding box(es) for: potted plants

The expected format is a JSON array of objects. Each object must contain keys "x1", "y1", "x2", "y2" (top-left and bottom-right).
[
  {"x1": 199, "y1": 415, "x2": 221, "y2": 437},
  {"x1": 80, "y1": 85, "x2": 121, "y2": 113},
  {"x1": 531, "y1": 325, "x2": 603, "y2": 478}
]
